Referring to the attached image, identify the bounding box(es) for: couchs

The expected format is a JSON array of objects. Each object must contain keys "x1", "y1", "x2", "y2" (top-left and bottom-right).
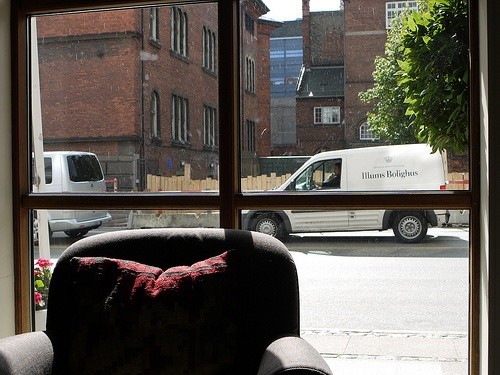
[{"x1": 0, "y1": 226, "x2": 333, "y2": 375}]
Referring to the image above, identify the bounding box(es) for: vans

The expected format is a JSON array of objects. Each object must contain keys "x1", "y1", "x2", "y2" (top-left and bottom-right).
[
  {"x1": 241, "y1": 143, "x2": 449, "y2": 244},
  {"x1": 32, "y1": 151, "x2": 112, "y2": 245}
]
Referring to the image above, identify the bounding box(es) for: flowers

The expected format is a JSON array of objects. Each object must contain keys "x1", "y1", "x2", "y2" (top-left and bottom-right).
[{"x1": 34, "y1": 257, "x2": 53, "y2": 311}]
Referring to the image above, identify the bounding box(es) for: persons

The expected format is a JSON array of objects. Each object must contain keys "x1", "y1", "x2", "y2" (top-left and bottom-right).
[{"x1": 311, "y1": 163, "x2": 341, "y2": 188}]
